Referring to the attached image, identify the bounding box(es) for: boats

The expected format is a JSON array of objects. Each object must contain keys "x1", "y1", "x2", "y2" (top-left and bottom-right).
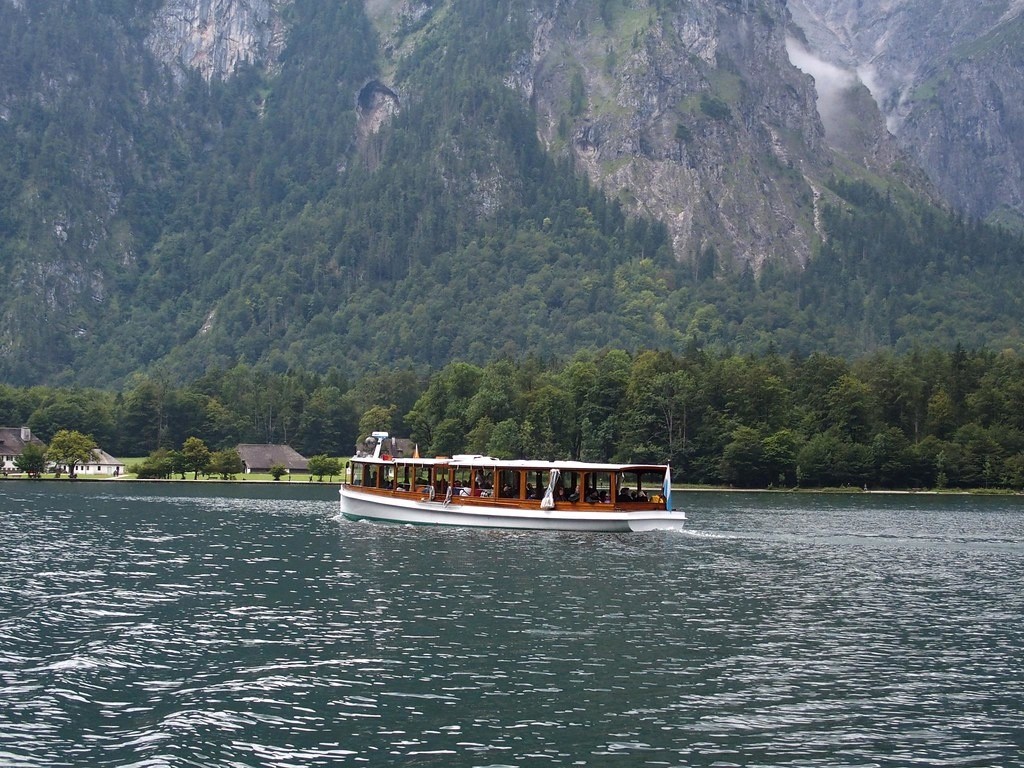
[{"x1": 338, "y1": 431, "x2": 689, "y2": 532}]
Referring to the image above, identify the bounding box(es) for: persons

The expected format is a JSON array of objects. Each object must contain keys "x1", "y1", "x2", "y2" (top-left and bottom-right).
[
  {"x1": 388, "y1": 480, "x2": 393, "y2": 489},
  {"x1": 371, "y1": 471, "x2": 377, "y2": 487},
  {"x1": 617, "y1": 488, "x2": 666, "y2": 503},
  {"x1": 554, "y1": 484, "x2": 580, "y2": 503},
  {"x1": 439, "y1": 469, "x2": 514, "y2": 499},
  {"x1": 526, "y1": 482, "x2": 545, "y2": 500},
  {"x1": 584, "y1": 487, "x2": 607, "y2": 504}
]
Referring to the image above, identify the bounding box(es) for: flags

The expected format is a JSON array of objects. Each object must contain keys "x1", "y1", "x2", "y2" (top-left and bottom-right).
[{"x1": 663, "y1": 463, "x2": 672, "y2": 514}]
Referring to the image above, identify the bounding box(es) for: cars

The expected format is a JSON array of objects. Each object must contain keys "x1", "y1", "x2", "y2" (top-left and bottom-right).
[{"x1": 49, "y1": 467, "x2": 67, "y2": 473}]
